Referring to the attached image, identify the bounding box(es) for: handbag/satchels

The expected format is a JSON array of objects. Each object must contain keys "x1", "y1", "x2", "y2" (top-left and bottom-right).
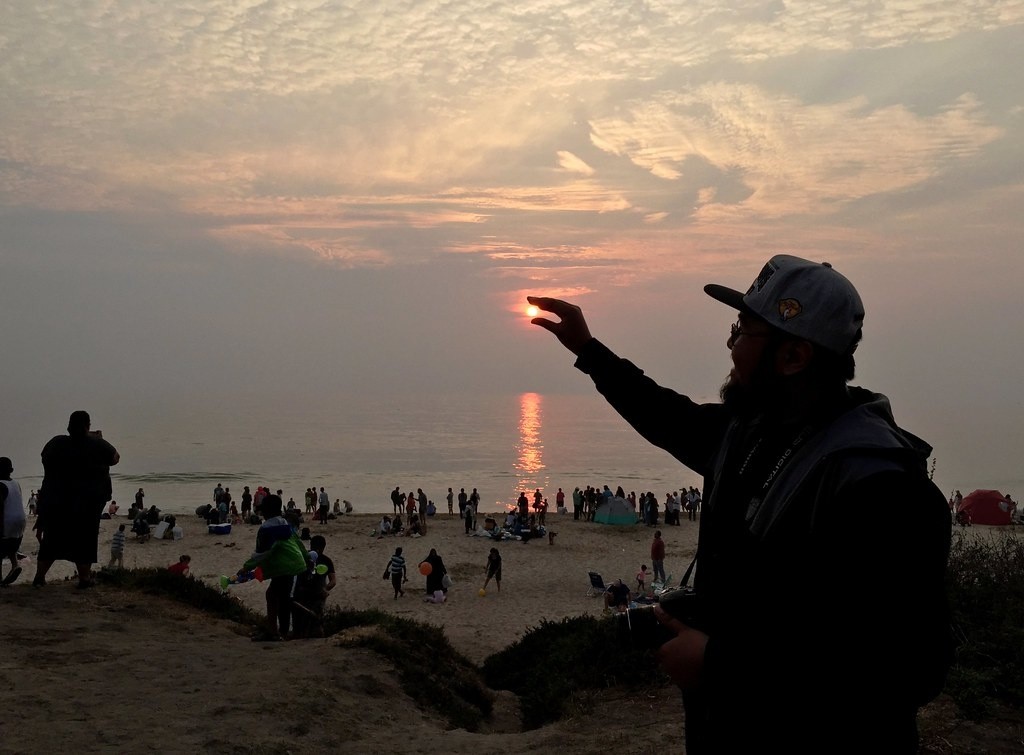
[{"x1": 441, "y1": 573, "x2": 453, "y2": 589}]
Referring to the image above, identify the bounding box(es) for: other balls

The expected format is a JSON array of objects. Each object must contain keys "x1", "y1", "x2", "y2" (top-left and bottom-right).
[
  {"x1": 479, "y1": 588, "x2": 486, "y2": 596},
  {"x1": 420, "y1": 562, "x2": 433, "y2": 575}
]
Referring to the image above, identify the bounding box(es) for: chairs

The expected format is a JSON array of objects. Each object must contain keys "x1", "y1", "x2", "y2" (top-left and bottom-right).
[
  {"x1": 131, "y1": 520, "x2": 151, "y2": 542},
  {"x1": 586, "y1": 571, "x2": 616, "y2": 597}
]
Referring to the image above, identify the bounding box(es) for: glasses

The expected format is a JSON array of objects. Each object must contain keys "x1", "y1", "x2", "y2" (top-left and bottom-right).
[{"x1": 730, "y1": 323, "x2": 761, "y2": 346}]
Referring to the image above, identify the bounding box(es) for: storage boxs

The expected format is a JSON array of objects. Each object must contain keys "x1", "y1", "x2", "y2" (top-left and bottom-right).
[
  {"x1": 513, "y1": 529, "x2": 531, "y2": 541},
  {"x1": 208, "y1": 523, "x2": 232, "y2": 534}
]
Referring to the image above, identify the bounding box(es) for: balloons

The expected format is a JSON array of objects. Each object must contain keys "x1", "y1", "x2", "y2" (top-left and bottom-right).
[{"x1": 478, "y1": 589, "x2": 486, "y2": 596}]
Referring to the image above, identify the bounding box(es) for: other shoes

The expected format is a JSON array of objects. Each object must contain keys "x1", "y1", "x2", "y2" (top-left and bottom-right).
[
  {"x1": 251, "y1": 632, "x2": 280, "y2": 642},
  {"x1": 77, "y1": 579, "x2": 92, "y2": 589},
  {"x1": 33, "y1": 578, "x2": 46, "y2": 588},
  {"x1": 1, "y1": 566, "x2": 22, "y2": 584}
]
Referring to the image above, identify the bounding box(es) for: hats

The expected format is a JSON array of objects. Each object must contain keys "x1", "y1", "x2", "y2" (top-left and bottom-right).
[{"x1": 703, "y1": 254, "x2": 865, "y2": 353}]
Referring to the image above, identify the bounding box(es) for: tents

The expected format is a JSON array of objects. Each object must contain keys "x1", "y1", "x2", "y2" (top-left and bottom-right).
[
  {"x1": 594, "y1": 496, "x2": 638, "y2": 526},
  {"x1": 955, "y1": 489, "x2": 1012, "y2": 526}
]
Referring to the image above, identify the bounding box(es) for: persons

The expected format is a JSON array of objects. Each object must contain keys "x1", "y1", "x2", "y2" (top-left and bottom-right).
[
  {"x1": 33, "y1": 410, "x2": 120, "y2": 589},
  {"x1": 607, "y1": 531, "x2": 665, "y2": 612},
  {"x1": 0, "y1": 457, "x2": 26, "y2": 585},
  {"x1": 528, "y1": 254, "x2": 963, "y2": 754},
  {"x1": 237, "y1": 494, "x2": 337, "y2": 641},
  {"x1": 196, "y1": 483, "x2": 353, "y2": 525},
  {"x1": 483, "y1": 547, "x2": 502, "y2": 592},
  {"x1": 27, "y1": 489, "x2": 43, "y2": 545},
  {"x1": 108, "y1": 488, "x2": 191, "y2": 578},
  {"x1": 417, "y1": 548, "x2": 448, "y2": 598},
  {"x1": 948, "y1": 490, "x2": 1019, "y2": 527},
  {"x1": 383, "y1": 547, "x2": 409, "y2": 601},
  {"x1": 379, "y1": 485, "x2": 702, "y2": 541}
]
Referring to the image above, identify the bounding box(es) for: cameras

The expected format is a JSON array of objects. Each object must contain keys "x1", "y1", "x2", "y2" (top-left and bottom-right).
[{"x1": 616, "y1": 589, "x2": 697, "y2": 654}]
